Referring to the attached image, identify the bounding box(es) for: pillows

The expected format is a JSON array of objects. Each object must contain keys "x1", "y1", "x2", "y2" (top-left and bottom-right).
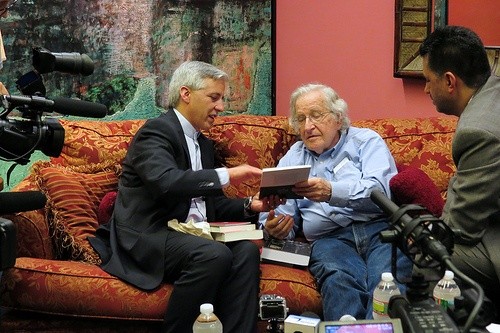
[
  {"x1": 27, "y1": 159, "x2": 120, "y2": 266},
  {"x1": 391, "y1": 164, "x2": 444, "y2": 218}
]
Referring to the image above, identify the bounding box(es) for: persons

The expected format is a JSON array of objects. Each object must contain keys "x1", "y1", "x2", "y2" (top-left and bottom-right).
[
  {"x1": 258, "y1": 83, "x2": 414, "y2": 321},
  {"x1": 418, "y1": 25, "x2": 500, "y2": 333},
  {"x1": 87, "y1": 62, "x2": 287, "y2": 333}
]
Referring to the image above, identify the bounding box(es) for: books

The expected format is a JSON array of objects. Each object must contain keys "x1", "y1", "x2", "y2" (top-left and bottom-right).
[
  {"x1": 259, "y1": 164, "x2": 311, "y2": 200},
  {"x1": 262, "y1": 239, "x2": 311, "y2": 266},
  {"x1": 203, "y1": 222, "x2": 263, "y2": 242}
]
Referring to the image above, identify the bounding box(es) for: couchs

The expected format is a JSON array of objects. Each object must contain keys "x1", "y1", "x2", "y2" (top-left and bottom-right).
[{"x1": 0, "y1": 114, "x2": 457, "y2": 333}]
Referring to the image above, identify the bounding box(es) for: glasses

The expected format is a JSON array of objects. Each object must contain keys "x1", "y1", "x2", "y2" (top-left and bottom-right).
[{"x1": 291, "y1": 110, "x2": 335, "y2": 126}]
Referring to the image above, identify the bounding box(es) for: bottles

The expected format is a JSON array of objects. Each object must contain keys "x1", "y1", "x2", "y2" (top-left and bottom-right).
[
  {"x1": 372, "y1": 272, "x2": 402, "y2": 321},
  {"x1": 432, "y1": 270, "x2": 462, "y2": 313},
  {"x1": 193, "y1": 303, "x2": 223, "y2": 333}
]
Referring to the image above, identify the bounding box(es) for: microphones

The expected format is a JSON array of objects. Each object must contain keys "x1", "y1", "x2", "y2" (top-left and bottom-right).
[
  {"x1": 0, "y1": 191, "x2": 48, "y2": 217},
  {"x1": 370, "y1": 190, "x2": 451, "y2": 261},
  {"x1": 51, "y1": 97, "x2": 107, "y2": 119}
]
